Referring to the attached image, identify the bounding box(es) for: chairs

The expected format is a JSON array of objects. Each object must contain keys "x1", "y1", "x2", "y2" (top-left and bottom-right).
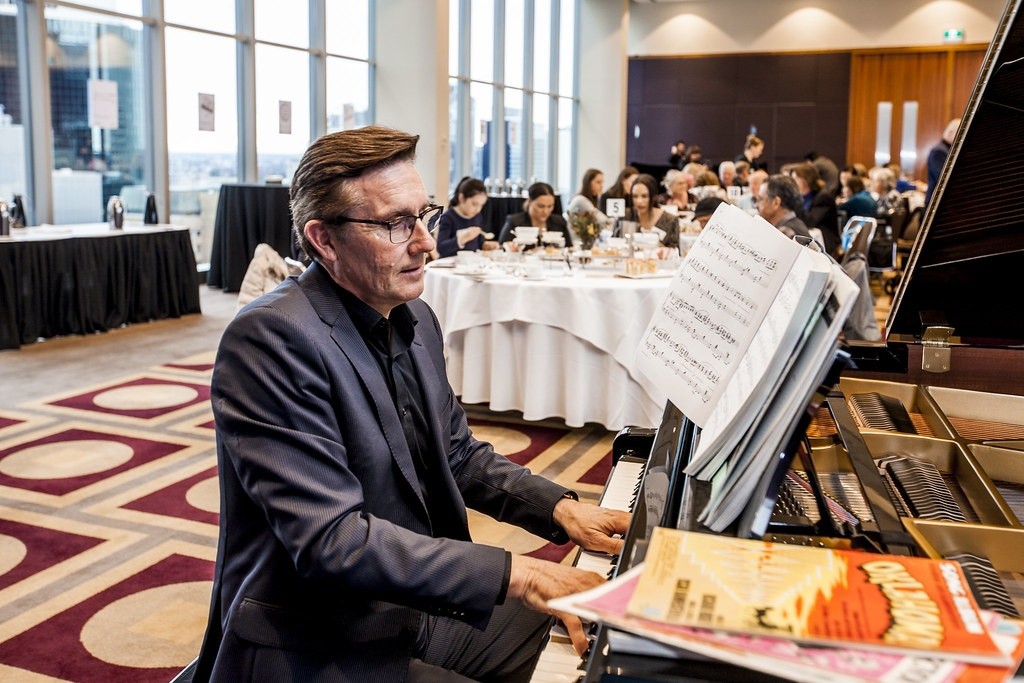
[{"x1": 841, "y1": 215, "x2": 878, "y2": 260}]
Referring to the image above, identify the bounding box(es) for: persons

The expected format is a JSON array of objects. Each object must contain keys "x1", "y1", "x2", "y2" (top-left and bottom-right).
[
  {"x1": 667, "y1": 141, "x2": 685, "y2": 170},
  {"x1": 683, "y1": 143, "x2": 712, "y2": 170},
  {"x1": 625, "y1": 174, "x2": 679, "y2": 249},
  {"x1": 657, "y1": 150, "x2": 928, "y2": 263},
  {"x1": 601, "y1": 166, "x2": 638, "y2": 216},
  {"x1": 194, "y1": 125, "x2": 631, "y2": 683},
  {"x1": 925, "y1": 116, "x2": 960, "y2": 210},
  {"x1": 567, "y1": 168, "x2": 609, "y2": 227},
  {"x1": 735, "y1": 135, "x2": 765, "y2": 170},
  {"x1": 436, "y1": 176, "x2": 501, "y2": 257},
  {"x1": 498, "y1": 181, "x2": 573, "y2": 248}
]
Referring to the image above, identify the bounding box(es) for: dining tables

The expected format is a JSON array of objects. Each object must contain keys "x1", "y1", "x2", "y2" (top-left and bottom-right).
[{"x1": 419, "y1": 245, "x2": 684, "y2": 432}]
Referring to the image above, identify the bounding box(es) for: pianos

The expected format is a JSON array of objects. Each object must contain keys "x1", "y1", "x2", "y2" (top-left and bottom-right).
[{"x1": 526, "y1": 0, "x2": 1022, "y2": 682}]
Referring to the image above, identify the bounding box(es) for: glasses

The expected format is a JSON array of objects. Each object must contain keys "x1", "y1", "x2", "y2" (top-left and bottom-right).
[{"x1": 325, "y1": 203, "x2": 444, "y2": 244}]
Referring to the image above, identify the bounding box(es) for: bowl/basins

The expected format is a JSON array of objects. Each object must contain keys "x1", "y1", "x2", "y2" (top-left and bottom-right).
[
  {"x1": 525, "y1": 265, "x2": 545, "y2": 277},
  {"x1": 457, "y1": 250, "x2": 473, "y2": 263},
  {"x1": 515, "y1": 227, "x2": 540, "y2": 240},
  {"x1": 542, "y1": 232, "x2": 562, "y2": 239}
]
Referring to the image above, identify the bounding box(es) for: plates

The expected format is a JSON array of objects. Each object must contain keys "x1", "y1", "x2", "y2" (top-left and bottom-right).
[
  {"x1": 514, "y1": 238, "x2": 538, "y2": 244},
  {"x1": 523, "y1": 276, "x2": 546, "y2": 280},
  {"x1": 541, "y1": 237, "x2": 564, "y2": 243},
  {"x1": 614, "y1": 271, "x2": 673, "y2": 278}
]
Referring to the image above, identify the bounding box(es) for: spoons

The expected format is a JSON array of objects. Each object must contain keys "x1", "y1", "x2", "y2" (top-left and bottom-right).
[{"x1": 480, "y1": 231, "x2": 494, "y2": 239}]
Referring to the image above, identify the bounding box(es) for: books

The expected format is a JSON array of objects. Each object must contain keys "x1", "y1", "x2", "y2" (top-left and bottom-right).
[
  {"x1": 635, "y1": 202, "x2": 858, "y2": 531},
  {"x1": 541, "y1": 528, "x2": 1024, "y2": 683}
]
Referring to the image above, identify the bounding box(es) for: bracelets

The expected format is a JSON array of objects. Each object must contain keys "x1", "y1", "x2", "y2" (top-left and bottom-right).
[{"x1": 559, "y1": 492, "x2": 574, "y2": 499}]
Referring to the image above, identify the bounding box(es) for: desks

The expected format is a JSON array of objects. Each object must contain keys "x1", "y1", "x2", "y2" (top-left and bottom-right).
[
  {"x1": 0, "y1": 221, "x2": 201, "y2": 351},
  {"x1": 475, "y1": 191, "x2": 562, "y2": 241},
  {"x1": 208, "y1": 181, "x2": 314, "y2": 293}
]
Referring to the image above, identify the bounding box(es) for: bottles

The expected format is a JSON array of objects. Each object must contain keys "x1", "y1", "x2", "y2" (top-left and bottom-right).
[
  {"x1": 108, "y1": 196, "x2": 125, "y2": 228},
  {"x1": 144, "y1": 195, "x2": 158, "y2": 223},
  {"x1": 0, "y1": 201, "x2": 9, "y2": 236},
  {"x1": 9, "y1": 194, "x2": 26, "y2": 228}
]
